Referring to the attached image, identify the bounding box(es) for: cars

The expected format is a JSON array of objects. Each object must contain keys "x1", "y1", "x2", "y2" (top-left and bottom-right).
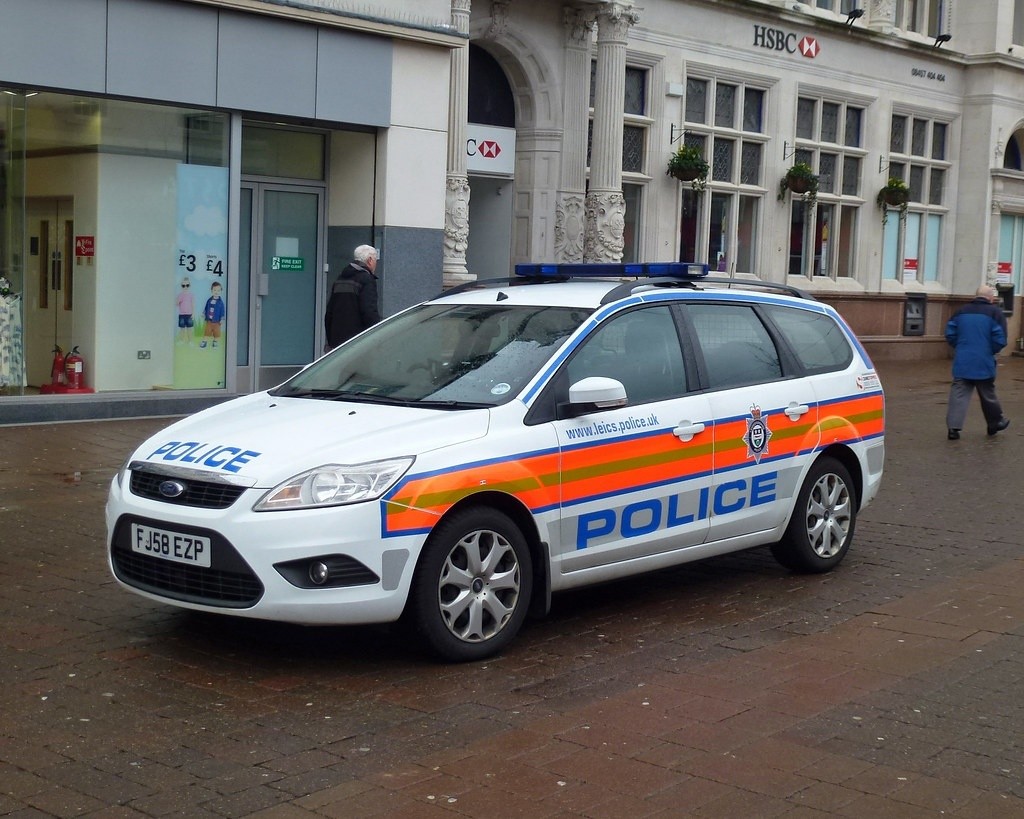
[{"x1": 105, "y1": 261, "x2": 887, "y2": 665}]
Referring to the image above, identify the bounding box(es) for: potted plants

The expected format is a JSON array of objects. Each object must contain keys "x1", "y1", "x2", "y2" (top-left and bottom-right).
[
  {"x1": 777, "y1": 162, "x2": 820, "y2": 225},
  {"x1": 877, "y1": 175, "x2": 911, "y2": 229},
  {"x1": 666, "y1": 146, "x2": 709, "y2": 193}
]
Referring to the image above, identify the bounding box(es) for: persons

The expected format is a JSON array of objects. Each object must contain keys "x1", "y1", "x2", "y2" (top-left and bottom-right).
[
  {"x1": 176, "y1": 276, "x2": 225, "y2": 348},
  {"x1": 324, "y1": 244, "x2": 381, "y2": 353},
  {"x1": 946, "y1": 286, "x2": 1010, "y2": 441}
]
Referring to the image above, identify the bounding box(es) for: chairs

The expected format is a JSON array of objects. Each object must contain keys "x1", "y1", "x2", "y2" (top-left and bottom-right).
[
  {"x1": 510, "y1": 325, "x2": 574, "y2": 409},
  {"x1": 618, "y1": 317, "x2": 669, "y2": 400}
]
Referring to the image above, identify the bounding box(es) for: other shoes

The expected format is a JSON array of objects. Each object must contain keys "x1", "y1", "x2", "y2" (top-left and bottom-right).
[
  {"x1": 987, "y1": 419, "x2": 1009, "y2": 435},
  {"x1": 948, "y1": 429, "x2": 960, "y2": 439}
]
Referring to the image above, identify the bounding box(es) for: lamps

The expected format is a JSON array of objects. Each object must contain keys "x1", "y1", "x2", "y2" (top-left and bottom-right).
[
  {"x1": 846, "y1": 9, "x2": 864, "y2": 27},
  {"x1": 934, "y1": 35, "x2": 952, "y2": 49}
]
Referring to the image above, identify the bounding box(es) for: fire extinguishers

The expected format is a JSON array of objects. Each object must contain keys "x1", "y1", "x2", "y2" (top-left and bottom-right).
[
  {"x1": 63, "y1": 345, "x2": 84, "y2": 390},
  {"x1": 50, "y1": 343, "x2": 65, "y2": 387}
]
